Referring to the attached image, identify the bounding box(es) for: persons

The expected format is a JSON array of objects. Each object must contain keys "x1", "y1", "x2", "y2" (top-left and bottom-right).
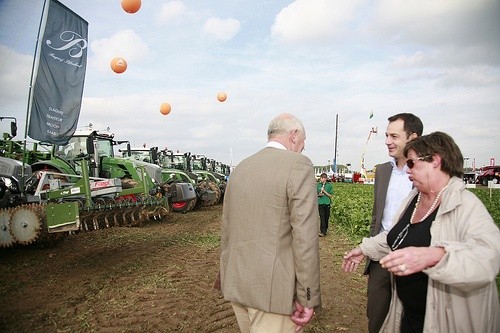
[
  {"x1": 363, "y1": 113, "x2": 424, "y2": 333},
  {"x1": 341, "y1": 131, "x2": 500, "y2": 333},
  {"x1": 317, "y1": 173, "x2": 335, "y2": 237},
  {"x1": 214, "y1": 114, "x2": 321, "y2": 333}
]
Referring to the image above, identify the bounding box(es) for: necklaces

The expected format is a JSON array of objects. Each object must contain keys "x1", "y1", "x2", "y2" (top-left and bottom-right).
[{"x1": 410, "y1": 186, "x2": 447, "y2": 224}]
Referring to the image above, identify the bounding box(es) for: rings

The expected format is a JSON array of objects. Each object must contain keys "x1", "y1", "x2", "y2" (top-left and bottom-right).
[{"x1": 400, "y1": 265, "x2": 406, "y2": 271}]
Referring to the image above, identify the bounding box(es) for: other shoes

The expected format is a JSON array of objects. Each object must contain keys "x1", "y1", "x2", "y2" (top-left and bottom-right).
[{"x1": 319, "y1": 234, "x2": 325, "y2": 237}]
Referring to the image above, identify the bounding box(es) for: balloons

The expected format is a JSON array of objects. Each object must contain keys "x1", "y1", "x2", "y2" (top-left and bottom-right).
[
  {"x1": 160, "y1": 103, "x2": 171, "y2": 115},
  {"x1": 110, "y1": 57, "x2": 128, "y2": 74},
  {"x1": 217, "y1": 91, "x2": 228, "y2": 102},
  {"x1": 120, "y1": 0, "x2": 142, "y2": 14}
]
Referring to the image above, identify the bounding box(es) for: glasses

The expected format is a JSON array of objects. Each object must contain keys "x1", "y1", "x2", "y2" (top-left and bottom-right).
[{"x1": 406, "y1": 153, "x2": 433, "y2": 169}]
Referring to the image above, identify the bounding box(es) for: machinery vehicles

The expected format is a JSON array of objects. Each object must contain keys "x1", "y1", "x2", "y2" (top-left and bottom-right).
[
  {"x1": 0, "y1": 116, "x2": 235, "y2": 248},
  {"x1": 477, "y1": 157, "x2": 500, "y2": 186}
]
